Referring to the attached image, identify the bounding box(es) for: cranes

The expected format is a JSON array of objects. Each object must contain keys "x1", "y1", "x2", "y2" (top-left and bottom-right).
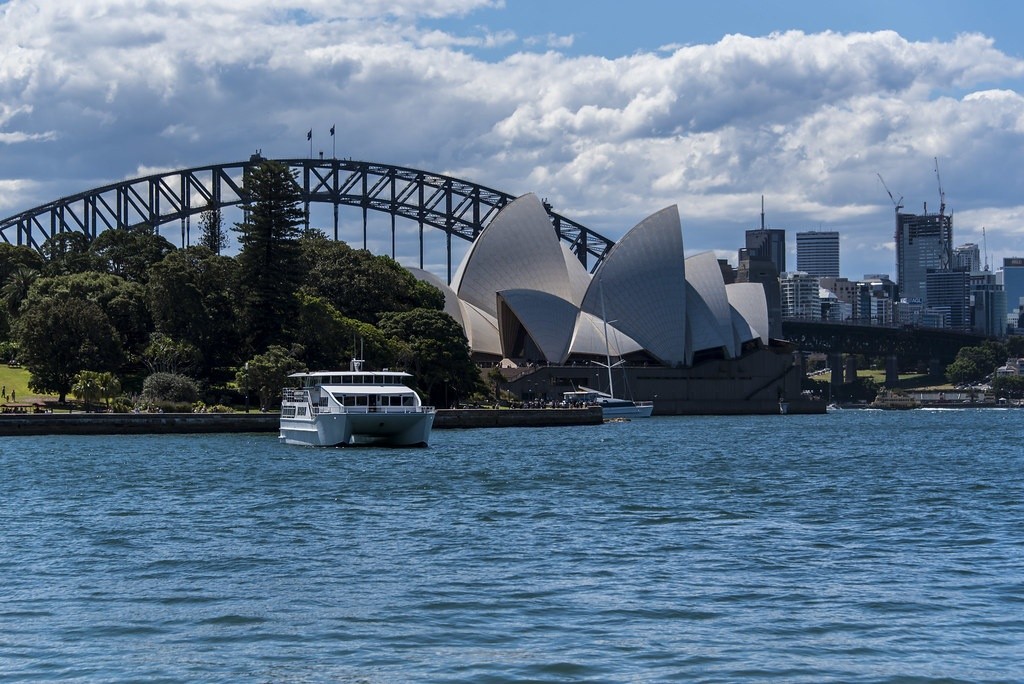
[
  {"x1": 877, "y1": 173, "x2": 905, "y2": 289},
  {"x1": 933, "y1": 156, "x2": 946, "y2": 273},
  {"x1": 984, "y1": 227, "x2": 989, "y2": 272}
]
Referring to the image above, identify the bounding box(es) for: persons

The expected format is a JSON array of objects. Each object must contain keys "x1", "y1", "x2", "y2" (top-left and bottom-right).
[
  {"x1": 450, "y1": 397, "x2": 594, "y2": 409},
  {"x1": 476, "y1": 360, "x2": 588, "y2": 368}
]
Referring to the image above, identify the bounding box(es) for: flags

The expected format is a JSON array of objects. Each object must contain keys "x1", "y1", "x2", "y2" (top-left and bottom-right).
[
  {"x1": 330, "y1": 126, "x2": 335, "y2": 136},
  {"x1": 308, "y1": 130, "x2": 311, "y2": 140}
]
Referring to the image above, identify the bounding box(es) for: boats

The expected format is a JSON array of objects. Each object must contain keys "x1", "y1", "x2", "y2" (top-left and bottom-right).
[
  {"x1": 870, "y1": 388, "x2": 923, "y2": 411},
  {"x1": 827, "y1": 404, "x2": 844, "y2": 412},
  {"x1": 278, "y1": 361, "x2": 442, "y2": 450}
]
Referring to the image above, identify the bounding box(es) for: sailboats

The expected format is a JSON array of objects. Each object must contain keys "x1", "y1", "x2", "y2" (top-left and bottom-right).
[{"x1": 564, "y1": 277, "x2": 653, "y2": 420}]
